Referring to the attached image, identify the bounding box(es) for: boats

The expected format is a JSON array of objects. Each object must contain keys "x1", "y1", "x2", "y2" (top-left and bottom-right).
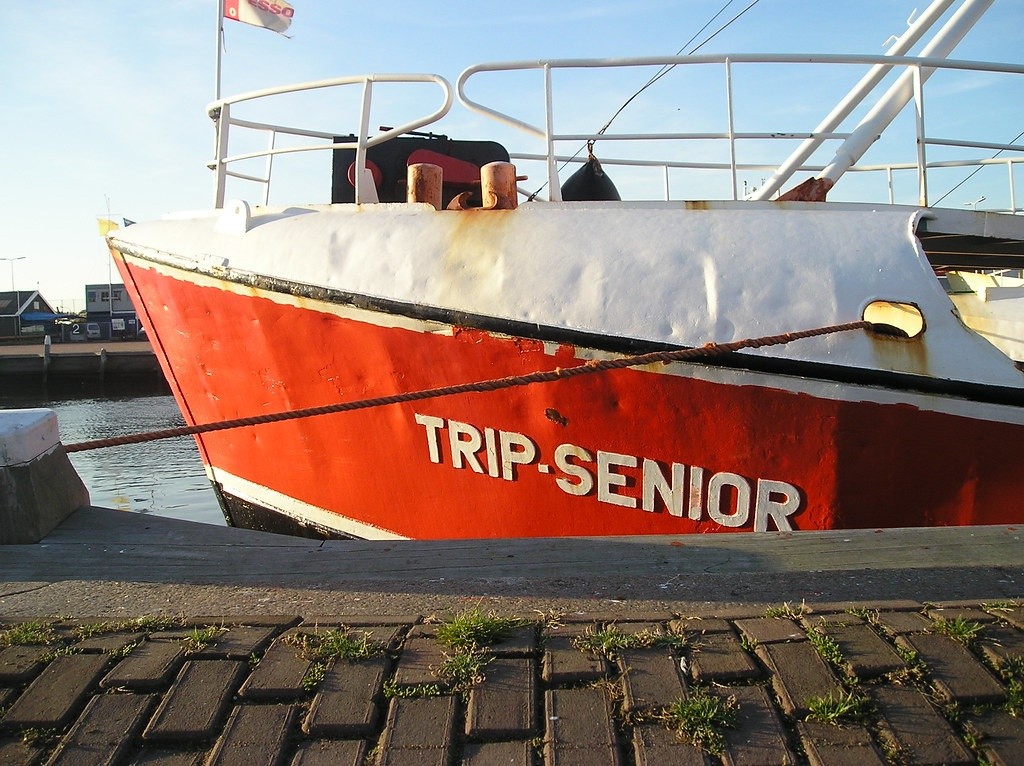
[{"x1": 103, "y1": 0, "x2": 1024, "y2": 542}]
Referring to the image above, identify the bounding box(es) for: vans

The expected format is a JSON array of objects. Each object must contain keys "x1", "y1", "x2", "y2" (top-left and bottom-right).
[{"x1": 77, "y1": 323, "x2": 101, "y2": 341}]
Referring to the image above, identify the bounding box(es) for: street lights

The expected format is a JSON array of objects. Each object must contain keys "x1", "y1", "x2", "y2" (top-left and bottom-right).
[{"x1": 0, "y1": 257, "x2": 27, "y2": 335}]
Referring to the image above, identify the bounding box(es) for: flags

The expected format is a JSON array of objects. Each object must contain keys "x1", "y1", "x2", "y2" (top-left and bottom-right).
[{"x1": 223, "y1": 0, "x2": 295, "y2": 33}]
